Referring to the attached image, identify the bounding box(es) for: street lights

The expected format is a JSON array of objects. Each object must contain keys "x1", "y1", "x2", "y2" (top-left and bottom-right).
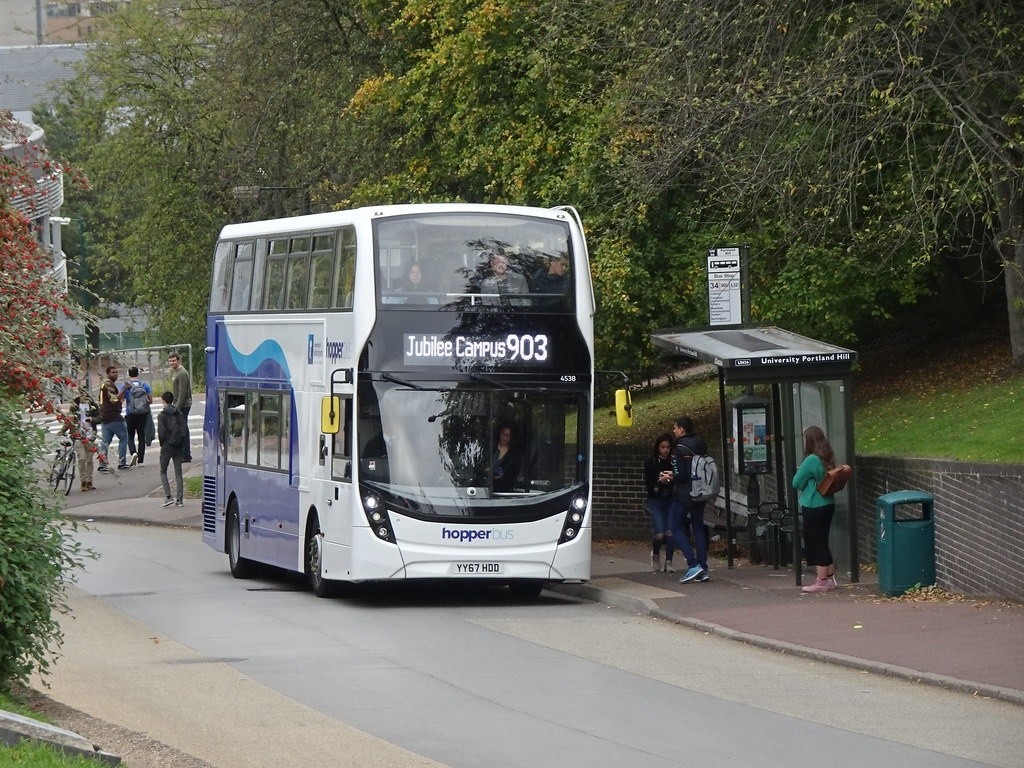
[{"x1": 233, "y1": 182, "x2": 312, "y2": 213}]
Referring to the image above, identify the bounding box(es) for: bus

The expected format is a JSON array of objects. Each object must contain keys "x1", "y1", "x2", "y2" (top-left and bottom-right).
[{"x1": 199, "y1": 202, "x2": 636, "y2": 600}]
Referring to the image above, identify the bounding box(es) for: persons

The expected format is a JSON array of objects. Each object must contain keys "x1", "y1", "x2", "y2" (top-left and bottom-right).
[
  {"x1": 493, "y1": 425, "x2": 519, "y2": 496},
  {"x1": 99, "y1": 367, "x2": 132, "y2": 471},
  {"x1": 158, "y1": 392, "x2": 184, "y2": 507},
  {"x1": 530, "y1": 251, "x2": 569, "y2": 308},
  {"x1": 59, "y1": 386, "x2": 103, "y2": 492},
  {"x1": 793, "y1": 426, "x2": 838, "y2": 593},
  {"x1": 481, "y1": 254, "x2": 532, "y2": 307},
  {"x1": 119, "y1": 367, "x2": 152, "y2": 466},
  {"x1": 397, "y1": 262, "x2": 439, "y2": 304},
  {"x1": 168, "y1": 352, "x2": 192, "y2": 461},
  {"x1": 665, "y1": 417, "x2": 710, "y2": 583},
  {"x1": 646, "y1": 433, "x2": 677, "y2": 574}
]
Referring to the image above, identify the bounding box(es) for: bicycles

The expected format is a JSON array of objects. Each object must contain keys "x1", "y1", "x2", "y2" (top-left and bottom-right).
[{"x1": 46, "y1": 433, "x2": 85, "y2": 497}]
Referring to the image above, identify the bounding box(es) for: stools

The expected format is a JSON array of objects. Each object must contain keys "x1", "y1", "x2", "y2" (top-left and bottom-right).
[{"x1": 756, "y1": 501, "x2": 806, "y2": 570}]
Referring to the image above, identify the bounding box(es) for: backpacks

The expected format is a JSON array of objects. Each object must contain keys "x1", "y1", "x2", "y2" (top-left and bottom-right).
[
  {"x1": 163, "y1": 412, "x2": 188, "y2": 448},
  {"x1": 813, "y1": 464, "x2": 852, "y2": 497},
  {"x1": 125, "y1": 381, "x2": 149, "y2": 415},
  {"x1": 677, "y1": 444, "x2": 720, "y2": 502}
]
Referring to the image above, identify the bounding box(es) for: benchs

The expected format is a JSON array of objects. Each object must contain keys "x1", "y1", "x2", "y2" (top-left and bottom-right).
[{"x1": 684, "y1": 495, "x2": 749, "y2": 557}]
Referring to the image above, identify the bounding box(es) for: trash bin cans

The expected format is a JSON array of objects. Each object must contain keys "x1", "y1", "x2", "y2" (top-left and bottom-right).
[{"x1": 877, "y1": 490, "x2": 936, "y2": 598}]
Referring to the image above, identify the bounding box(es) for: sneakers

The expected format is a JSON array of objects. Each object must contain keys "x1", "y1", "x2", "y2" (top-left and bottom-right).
[
  {"x1": 160, "y1": 495, "x2": 174, "y2": 508},
  {"x1": 828, "y1": 575, "x2": 838, "y2": 589},
  {"x1": 693, "y1": 569, "x2": 710, "y2": 582},
  {"x1": 175, "y1": 500, "x2": 183, "y2": 507},
  {"x1": 678, "y1": 563, "x2": 704, "y2": 582},
  {"x1": 802, "y1": 578, "x2": 828, "y2": 593}
]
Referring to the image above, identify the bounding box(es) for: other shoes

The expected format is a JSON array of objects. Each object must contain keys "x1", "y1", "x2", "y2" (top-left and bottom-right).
[
  {"x1": 129, "y1": 453, "x2": 138, "y2": 467},
  {"x1": 651, "y1": 551, "x2": 661, "y2": 571},
  {"x1": 87, "y1": 481, "x2": 96, "y2": 490},
  {"x1": 80, "y1": 482, "x2": 87, "y2": 491},
  {"x1": 664, "y1": 560, "x2": 675, "y2": 572},
  {"x1": 98, "y1": 466, "x2": 111, "y2": 471},
  {"x1": 138, "y1": 462, "x2": 146, "y2": 467},
  {"x1": 118, "y1": 464, "x2": 132, "y2": 469},
  {"x1": 180, "y1": 454, "x2": 192, "y2": 463}
]
